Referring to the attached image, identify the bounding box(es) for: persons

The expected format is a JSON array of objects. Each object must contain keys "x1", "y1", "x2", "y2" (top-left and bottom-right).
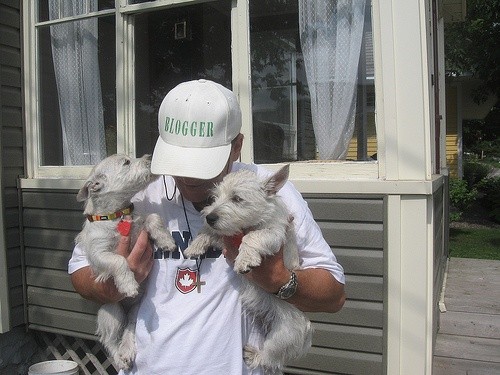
[{"x1": 68, "y1": 78, "x2": 345, "y2": 375}]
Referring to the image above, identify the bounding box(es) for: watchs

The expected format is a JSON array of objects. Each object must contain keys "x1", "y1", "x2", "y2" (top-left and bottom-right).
[{"x1": 271, "y1": 269, "x2": 298, "y2": 301}]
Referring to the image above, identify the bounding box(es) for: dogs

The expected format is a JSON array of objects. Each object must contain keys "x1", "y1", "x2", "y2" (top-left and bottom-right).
[
  {"x1": 182, "y1": 163, "x2": 312, "y2": 375},
  {"x1": 76, "y1": 153, "x2": 177, "y2": 373}
]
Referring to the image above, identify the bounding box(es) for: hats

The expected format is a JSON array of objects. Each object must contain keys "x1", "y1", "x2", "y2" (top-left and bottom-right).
[{"x1": 147, "y1": 76, "x2": 243, "y2": 180}]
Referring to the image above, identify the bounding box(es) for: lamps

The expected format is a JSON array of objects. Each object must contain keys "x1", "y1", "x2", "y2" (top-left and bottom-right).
[{"x1": 174, "y1": 7, "x2": 192, "y2": 42}]
